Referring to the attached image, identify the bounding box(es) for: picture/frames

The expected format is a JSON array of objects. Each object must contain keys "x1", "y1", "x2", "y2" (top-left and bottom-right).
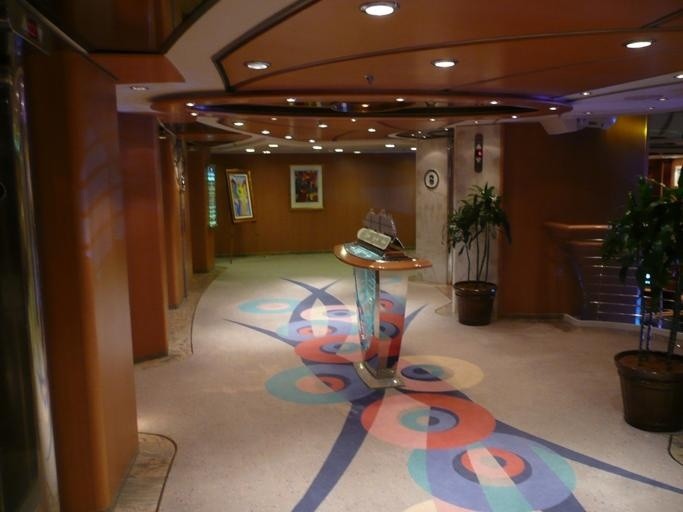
[
  {"x1": 225, "y1": 168, "x2": 256, "y2": 223},
  {"x1": 288, "y1": 162, "x2": 326, "y2": 211}
]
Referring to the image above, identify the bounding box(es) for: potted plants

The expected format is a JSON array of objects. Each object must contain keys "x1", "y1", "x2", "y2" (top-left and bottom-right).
[
  {"x1": 601, "y1": 166, "x2": 683, "y2": 433},
  {"x1": 441, "y1": 181, "x2": 512, "y2": 326}
]
